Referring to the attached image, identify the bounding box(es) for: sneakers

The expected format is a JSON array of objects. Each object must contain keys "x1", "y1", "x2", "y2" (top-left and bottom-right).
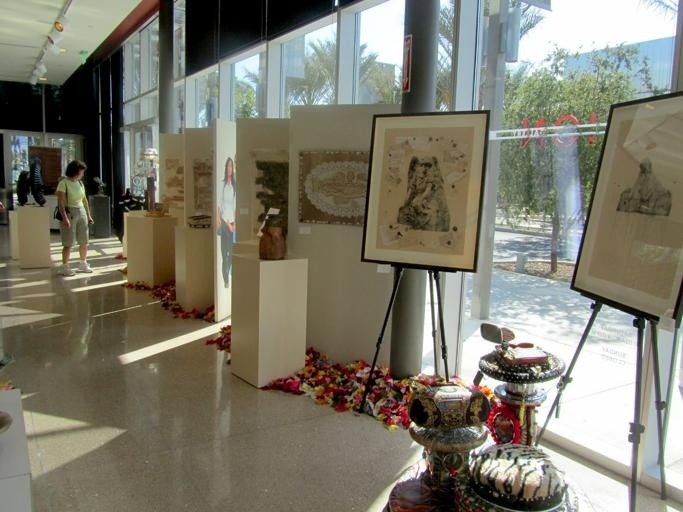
[
  {"x1": 60, "y1": 264, "x2": 76, "y2": 276},
  {"x1": 79, "y1": 262, "x2": 93, "y2": 272}
]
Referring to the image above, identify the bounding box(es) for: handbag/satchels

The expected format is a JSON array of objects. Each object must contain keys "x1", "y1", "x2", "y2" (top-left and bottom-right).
[{"x1": 56, "y1": 207, "x2": 68, "y2": 221}]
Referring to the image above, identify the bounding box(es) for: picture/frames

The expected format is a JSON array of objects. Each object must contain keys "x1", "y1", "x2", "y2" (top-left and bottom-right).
[
  {"x1": 570, "y1": 92, "x2": 683, "y2": 324},
  {"x1": 361, "y1": 111, "x2": 489, "y2": 272}
]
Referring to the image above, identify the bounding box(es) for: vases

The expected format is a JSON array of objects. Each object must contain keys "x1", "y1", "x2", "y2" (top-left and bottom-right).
[{"x1": 260, "y1": 226, "x2": 286, "y2": 260}]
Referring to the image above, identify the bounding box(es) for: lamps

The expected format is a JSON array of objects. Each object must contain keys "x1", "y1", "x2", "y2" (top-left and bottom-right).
[
  {"x1": 47, "y1": 33, "x2": 63, "y2": 46},
  {"x1": 55, "y1": 14, "x2": 69, "y2": 32},
  {"x1": 28, "y1": 59, "x2": 48, "y2": 85}
]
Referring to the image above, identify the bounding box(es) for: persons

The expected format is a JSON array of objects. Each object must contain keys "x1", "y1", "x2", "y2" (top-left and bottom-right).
[
  {"x1": 16, "y1": 169, "x2": 32, "y2": 205},
  {"x1": 216, "y1": 154, "x2": 237, "y2": 288},
  {"x1": 56, "y1": 161, "x2": 94, "y2": 276}
]
